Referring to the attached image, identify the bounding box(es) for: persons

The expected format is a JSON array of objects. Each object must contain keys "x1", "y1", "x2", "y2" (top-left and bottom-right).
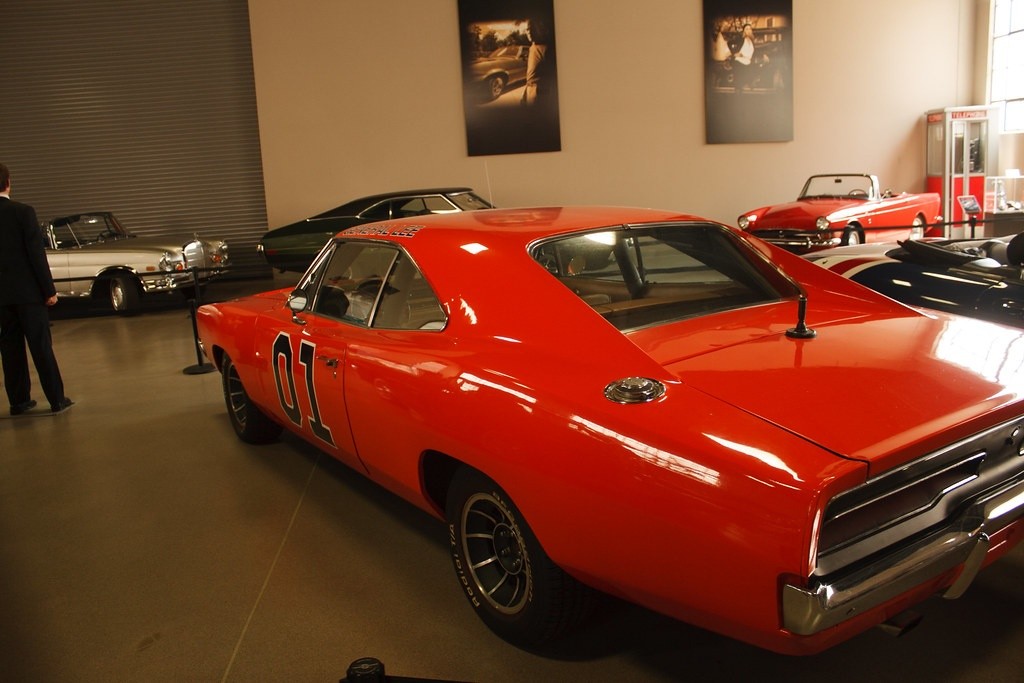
[
  {"x1": 518, "y1": 18, "x2": 556, "y2": 109},
  {"x1": 726, "y1": 21, "x2": 771, "y2": 97},
  {"x1": 0, "y1": 164, "x2": 76, "y2": 415}
]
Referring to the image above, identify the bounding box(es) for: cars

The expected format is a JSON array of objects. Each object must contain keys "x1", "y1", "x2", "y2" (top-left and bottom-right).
[
  {"x1": 461, "y1": 43, "x2": 534, "y2": 102},
  {"x1": 195, "y1": 206, "x2": 1024, "y2": 659},
  {"x1": 256, "y1": 185, "x2": 617, "y2": 281},
  {"x1": 42, "y1": 211, "x2": 231, "y2": 318},
  {"x1": 795, "y1": 229, "x2": 1024, "y2": 332},
  {"x1": 736, "y1": 171, "x2": 941, "y2": 257}
]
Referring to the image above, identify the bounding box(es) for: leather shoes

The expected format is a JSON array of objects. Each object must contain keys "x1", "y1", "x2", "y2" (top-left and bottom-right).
[
  {"x1": 10, "y1": 400, "x2": 37, "y2": 415},
  {"x1": 51, "y1": 397, "x2": 74, "y2": 414}
]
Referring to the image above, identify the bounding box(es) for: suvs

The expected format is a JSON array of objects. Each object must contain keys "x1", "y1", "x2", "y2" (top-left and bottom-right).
[{"x1": 716, "y1": 26, "x2": 786, "y2": 88}]
